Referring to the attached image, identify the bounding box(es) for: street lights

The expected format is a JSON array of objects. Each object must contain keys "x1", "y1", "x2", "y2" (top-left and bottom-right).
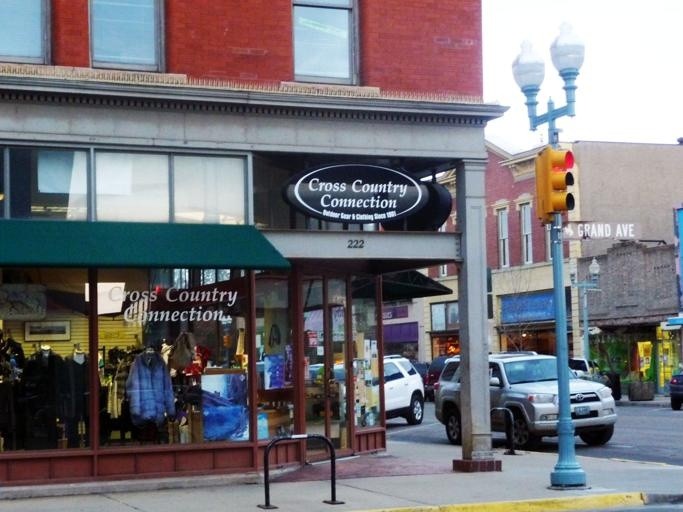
[
  {"x1": 512, "y1": 30, "x2": 585, "y2": 488},
  {"x1": 569, "y1": 255, "x2": 599, "y2": 362}
]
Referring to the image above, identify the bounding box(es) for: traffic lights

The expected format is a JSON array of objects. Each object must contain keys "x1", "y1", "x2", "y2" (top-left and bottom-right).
[{"x1": 534, "y1": 144, "x2": 574, "y2": 224}]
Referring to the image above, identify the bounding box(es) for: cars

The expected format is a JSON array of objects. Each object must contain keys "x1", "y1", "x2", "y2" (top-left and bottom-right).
[
  {"x1": 411, "y1": 360, "x2": 429, "y2": 385},
  {"x1": 173, "y1": 383, "x2": 198, "y2": 401},
  {"x1": 307, "y1": 362, "x2": 335, "y2": 374},
  {"x1": 670, "y1": 362, "x2": 683, "y2": 411}
]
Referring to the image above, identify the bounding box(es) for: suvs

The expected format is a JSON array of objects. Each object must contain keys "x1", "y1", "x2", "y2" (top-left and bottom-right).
[
  {"x1": 334, "y1": 355, "x2": 426, "y2": 429},
  {"x1": 424, "y1": 354, "x2": 457, "y2": 402},
  {"x1": 433, "y1": 349, "x2": 617, "y2": 451},
  {"x1": 568, "y1": 358, "x2": 600, "y2": 379}
]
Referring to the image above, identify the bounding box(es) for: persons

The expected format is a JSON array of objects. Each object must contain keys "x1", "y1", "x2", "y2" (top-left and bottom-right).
[
  {"x1": 126, "y1": 350, "x2": 176, "y2": 425},
  {"x1": 58, "y1": 348, "x2": 89, "y2": 447},
  {"x1": 22, "y1": 344, "x2": 66, "y2": 448}
]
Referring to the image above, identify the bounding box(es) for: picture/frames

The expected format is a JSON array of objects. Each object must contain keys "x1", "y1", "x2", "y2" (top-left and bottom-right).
[{"x1": 23, "y1": 319, "x2": 72, "y2": 342}]
